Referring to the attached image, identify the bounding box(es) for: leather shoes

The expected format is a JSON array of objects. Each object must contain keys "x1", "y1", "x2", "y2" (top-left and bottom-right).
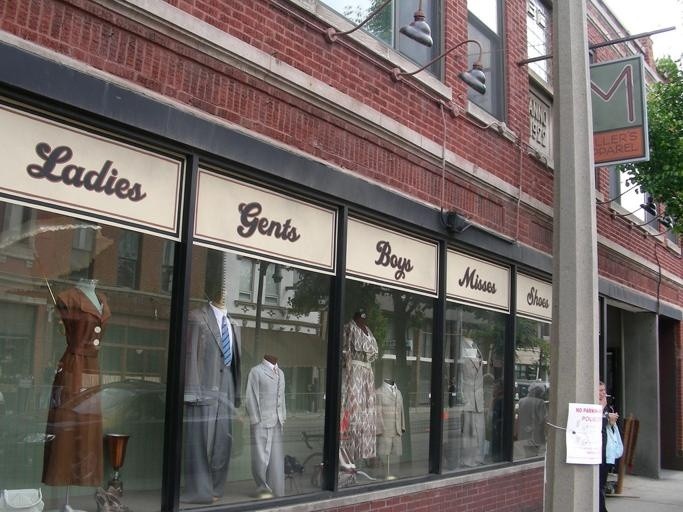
[
  {"x1": 96, "y1": 487, "x2": 129, "y2": 512},
  {"x1": 284, "y1": 455, "x2": 304, "y2": 474}
]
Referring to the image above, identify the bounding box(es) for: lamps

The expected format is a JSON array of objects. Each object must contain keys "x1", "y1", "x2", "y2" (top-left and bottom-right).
[
  {"x1": 391, "y1": 40, "x2": 487, "y2": 95},
  {"x1": 326, "y1": 0, "x2": 433, "y2": 48},
  {"x1": 596, "y1": 178, "x2": 678, "y2": 238}
]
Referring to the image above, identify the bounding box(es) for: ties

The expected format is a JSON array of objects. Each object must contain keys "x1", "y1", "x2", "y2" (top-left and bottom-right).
[
  {"x1": 222, "y1": 316, "x2": 232, "y2": 367},
  {"x1": 273, "y1": 367, "x2": 278, "y2": 375},
  {"x1": 391, "y1": 387, "x2": 395, "y2": 395}
]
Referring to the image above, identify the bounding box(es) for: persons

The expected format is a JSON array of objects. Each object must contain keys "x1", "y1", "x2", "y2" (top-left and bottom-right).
[
  {"x1": 457, "y1": 337, "x2": 487, "y2": 467},
  {"x1": 39, "y1": 277, "x2": 113, "y2": 486},
  {"x1": 179, "y1": 291, "x2": 243, "y2": 505},
  {"x1": 488, "y1": 343, "x2": 504, "y2": 463},
  {"x1": 375, "y1": 378, "x2": 405, "y2": 456},
  {"x1": 461, "y1": 373, "x2": 548, "y2": 459},
  {"x1": 244, "y1": 354, "x2": 288, "y2": 500},
  {"x1": 339, "y1": 312, "x2": 379, "y2": 459},
  {"x1": 599, "y1": 380, "x2": 620, "y2": 511}
]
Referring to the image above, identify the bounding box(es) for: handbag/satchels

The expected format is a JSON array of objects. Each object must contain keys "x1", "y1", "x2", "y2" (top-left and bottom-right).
[
  {"x1": 606, "y1": 424, "x2": 623, "y2": 464},
  {"x1": 312, "y1": 464, "x2": 357, "y2": 489},
  {"x1": 0, "y1": 489, "x2": 44, "y2": 512}
]
